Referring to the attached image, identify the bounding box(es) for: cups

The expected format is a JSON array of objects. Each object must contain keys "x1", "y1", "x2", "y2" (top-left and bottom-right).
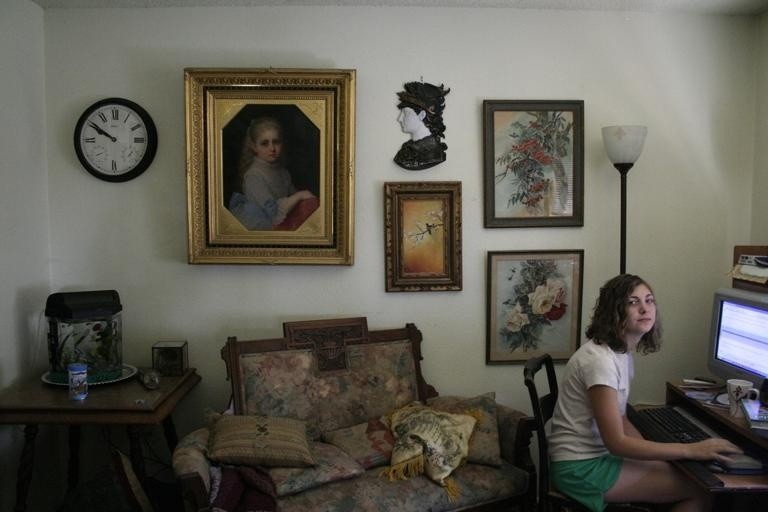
[{"x1": 726, "y1": 378, "x2": 761, "y2": 418}]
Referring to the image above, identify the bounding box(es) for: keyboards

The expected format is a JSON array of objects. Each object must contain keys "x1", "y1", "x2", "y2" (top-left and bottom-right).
[{"x1": 637, "y1": 406, "x2": 713, "y2": 444}]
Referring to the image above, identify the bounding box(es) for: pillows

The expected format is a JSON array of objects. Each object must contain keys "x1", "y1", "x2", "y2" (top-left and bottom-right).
[
  {"x1": 261, "y1": 442, "x2": 365, "y2": 497},
  {"x1": 206, "y1": 412, "x2": 319, "y2": 466},
  {"x1": 425, "y1": 391, "x2": 503, "y2": 466},
  {"x1": 321, "y1": 415, "x2": 398, "y2": 470}
]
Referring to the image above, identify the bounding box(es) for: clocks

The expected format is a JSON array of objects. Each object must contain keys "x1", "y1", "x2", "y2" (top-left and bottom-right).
[{"x1": 74, "y1": 98, "x2": 157, "y2": 183}]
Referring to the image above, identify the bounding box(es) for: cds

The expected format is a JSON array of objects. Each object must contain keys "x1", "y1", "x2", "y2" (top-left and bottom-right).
[
  {"x1": 685, "y1": 391, "x2": 714, "y2": 401},
  {"x1": 716, "y1": 393, "x2": 730, "y2": 405}
]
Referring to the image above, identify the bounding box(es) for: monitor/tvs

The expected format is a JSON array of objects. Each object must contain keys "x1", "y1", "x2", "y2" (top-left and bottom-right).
[{"x1": 709, "y1": 288, "x2": 768, "y2": 380}]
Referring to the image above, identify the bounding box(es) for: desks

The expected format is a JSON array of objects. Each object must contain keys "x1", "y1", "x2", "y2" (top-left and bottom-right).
[
  {"x1": 628, "y1": 378, "x2": 768, "y2": 512},
  {"x1": 0, "y1": 365, "x2": 202, "y2": 512}
]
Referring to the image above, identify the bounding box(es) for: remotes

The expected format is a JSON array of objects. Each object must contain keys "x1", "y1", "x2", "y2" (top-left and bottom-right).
[{"x1": 681, "y1": 379, "x2": 713, "y2": 385}]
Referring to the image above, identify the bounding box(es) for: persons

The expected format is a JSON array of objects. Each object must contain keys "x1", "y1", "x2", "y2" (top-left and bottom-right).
[
  {"x1": 394, "y1": 82, "x2": 447, "y2": 169},
  {"x1": 547, "y1": 274, "x2": 743, "y2": 512},
  {"x1": 238, "y1": 116, "x2": 316, "y2": 230}
]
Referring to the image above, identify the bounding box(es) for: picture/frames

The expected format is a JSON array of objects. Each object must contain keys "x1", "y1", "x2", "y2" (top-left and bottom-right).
[
  {"x1": 482, "y1": 99, "x2": 585, "y2": 230},
  {"x1": 184, "y1": 67, "x2": 355, "y2": 266},
  {"x1": 384, "y1": 181, "x2": 463, "y2": 292},
  {"x1": 486, "y1": 248, "x2": 584, "y2": 365}
]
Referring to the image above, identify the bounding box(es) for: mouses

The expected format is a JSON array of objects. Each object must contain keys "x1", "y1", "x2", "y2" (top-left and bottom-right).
[{"x1": 723, "y1": 454, "x2": 762, "y2": 469}]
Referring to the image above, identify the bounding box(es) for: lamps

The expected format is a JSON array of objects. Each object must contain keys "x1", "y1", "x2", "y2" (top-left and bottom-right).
[{"x1": 602, "y1": 125, "x2": 648, "y2": 276}]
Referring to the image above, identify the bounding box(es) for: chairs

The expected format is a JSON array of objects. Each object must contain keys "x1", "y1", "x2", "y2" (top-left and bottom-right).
[
  {"x1": 524, "y1": 352, "x2": 650, "y2": 512},
  {"x1": 171, "y1": 316, "x2": 539, "y2": 512}
]
